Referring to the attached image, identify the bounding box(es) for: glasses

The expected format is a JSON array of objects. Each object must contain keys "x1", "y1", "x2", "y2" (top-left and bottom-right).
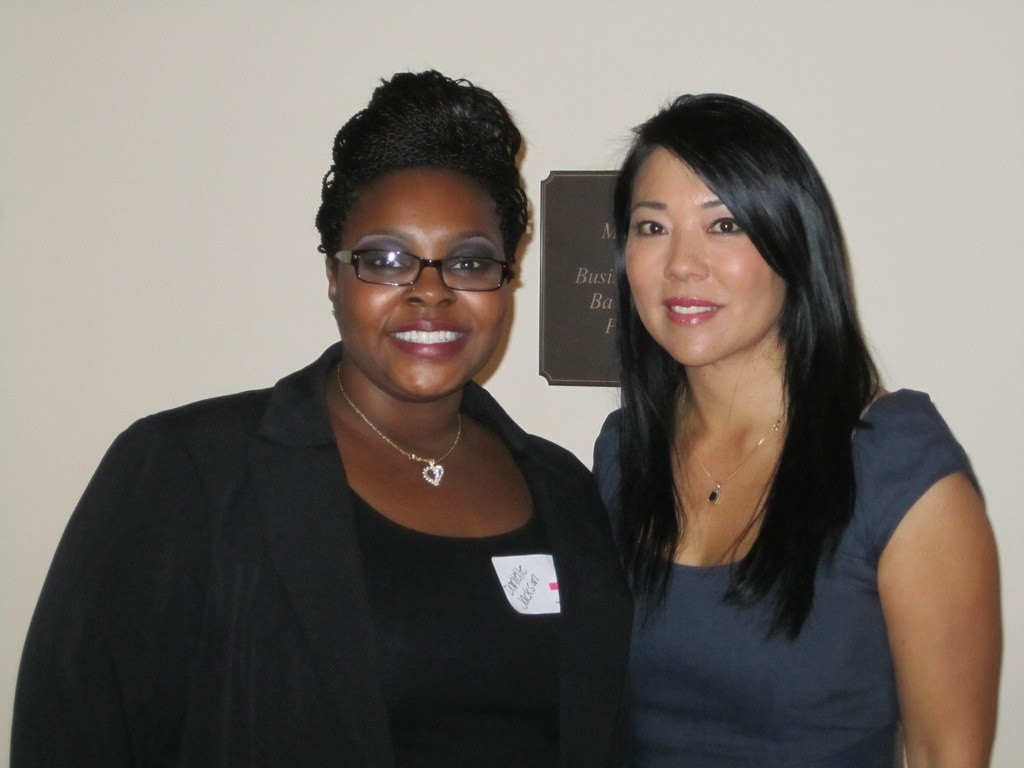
[{"x1": 334, "y1": 248, "x2": 511, "y2": 292}]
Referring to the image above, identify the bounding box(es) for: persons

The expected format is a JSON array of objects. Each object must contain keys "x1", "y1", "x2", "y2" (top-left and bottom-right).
[
  {"x1": 592, "y1": 95, "x2": 1003, "y2": 768},
  {"x1": 11, "y1": 71, "x2": 634, "y2": 768}
]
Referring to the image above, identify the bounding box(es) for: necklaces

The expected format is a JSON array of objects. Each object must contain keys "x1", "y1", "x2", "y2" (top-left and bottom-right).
[
  {"x1": 336, "y1": 360, "x2": 461, "y2": 486},
  {"x1": 685, "y1": 413, "x2": 784, "y2": 505}
]
